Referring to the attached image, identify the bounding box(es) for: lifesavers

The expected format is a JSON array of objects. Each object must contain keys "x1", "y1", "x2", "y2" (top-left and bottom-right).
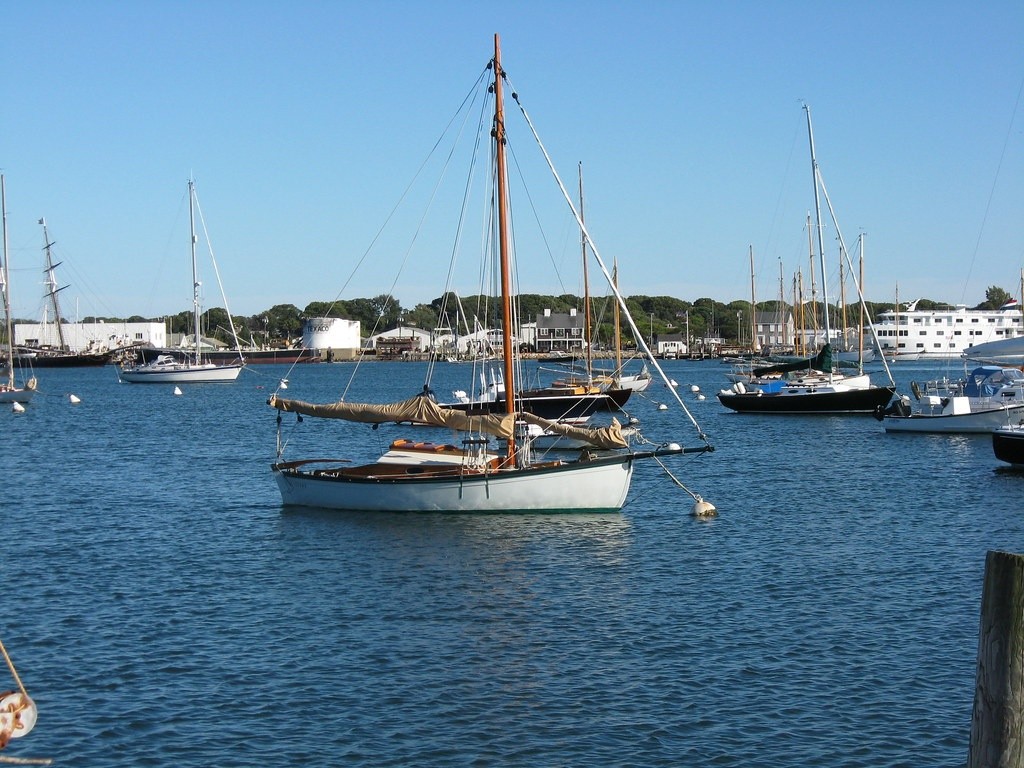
[{"x1": 911, "y1": 380, "x2": 922, "y2": 400}]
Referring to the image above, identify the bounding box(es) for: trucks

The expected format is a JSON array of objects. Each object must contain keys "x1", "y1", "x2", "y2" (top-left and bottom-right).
[{"x1": 657, "y1": 340, "x2": 688, "y2": 354}]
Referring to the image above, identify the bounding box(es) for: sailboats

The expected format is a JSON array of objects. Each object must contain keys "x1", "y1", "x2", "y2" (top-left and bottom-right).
[
  {"x1": 715, "y1": 100, "x2": 1024, "y2": 471},
  {"x1": 0, "y1": 216, "x2": 149, "y2": 368},
  {"x1": 262, "y1": 30, "x2": 714, "y2": 517},
  {"x1": 119, "y1": 179, "x2": 247, "y2": 384},
  {"x1": 0, "y1": 173, "x2": 39, "y2": 403}
]
